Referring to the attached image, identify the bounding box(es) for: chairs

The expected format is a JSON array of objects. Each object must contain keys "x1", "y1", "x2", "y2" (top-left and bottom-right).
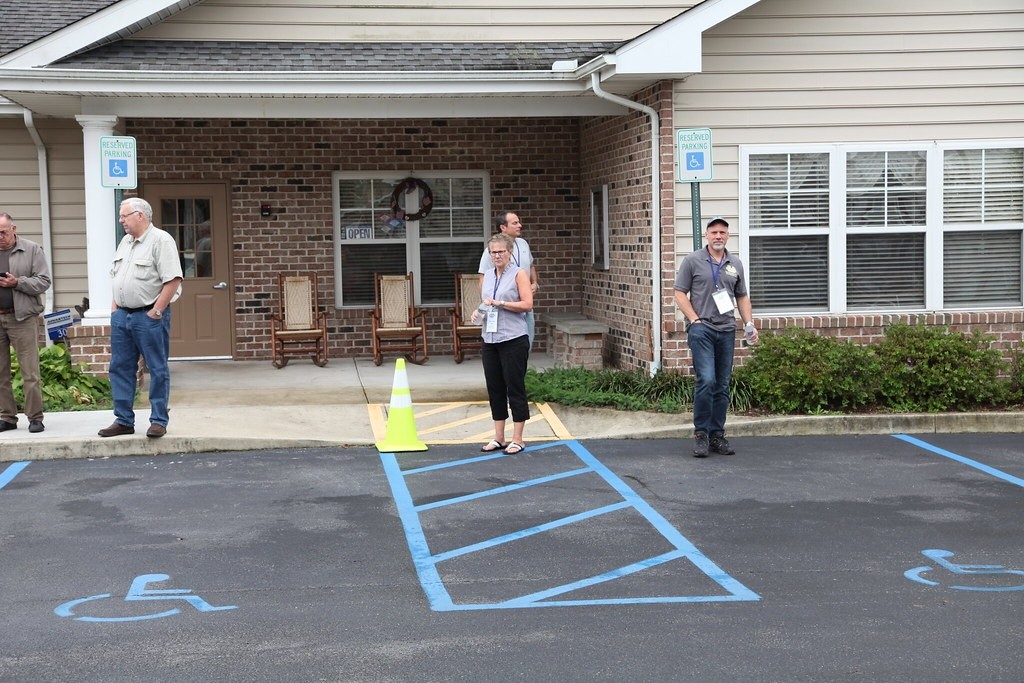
[
  {"x1": 368, "y1": 271, "x2": 430, "y2": 366},
  {"x1": 448, "y1": 272, "x2": 485, "y2": 364},
  {"x1": 270, "y1": 271, "x2": 330, "y2": 370}
]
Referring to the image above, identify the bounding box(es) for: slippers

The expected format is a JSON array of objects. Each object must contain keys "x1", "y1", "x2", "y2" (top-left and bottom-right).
[
  {"x1": 482, "y1": 439, "x2": 507, "y2": 451},
  {"x1": 503, "y1": 443, "x2": 524, "y2": 455}
]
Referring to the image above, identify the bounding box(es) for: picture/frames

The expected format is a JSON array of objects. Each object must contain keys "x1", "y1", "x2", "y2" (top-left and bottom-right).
[{"x1": 589, "y1": 185, "x2": 610, "y2": 270}]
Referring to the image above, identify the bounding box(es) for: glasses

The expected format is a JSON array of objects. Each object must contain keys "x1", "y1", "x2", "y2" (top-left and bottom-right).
[
  {"x1": 118, "y1": 211, "x2": 138, "y2": 219},
  {"x1": 490, "y1": 249, "x2": 508, "y2": 257}
]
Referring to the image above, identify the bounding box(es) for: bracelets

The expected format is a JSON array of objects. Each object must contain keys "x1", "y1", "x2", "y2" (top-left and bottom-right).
[
  {"x1": 745, "y1": 322, "x2": 755, "y2": 327},
  {"x1": 692, "y1": 319, "x2": 700, "y2": 324}
]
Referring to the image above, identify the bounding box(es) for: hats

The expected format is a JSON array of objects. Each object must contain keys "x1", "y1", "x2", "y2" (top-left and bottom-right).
[{"x1": 706, "y1": 216, "x2": 729, "y2": 233}]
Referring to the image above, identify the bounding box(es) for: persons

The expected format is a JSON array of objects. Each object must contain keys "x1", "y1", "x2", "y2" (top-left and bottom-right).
[
  {"x1": 675, "y1": 216, "x2": 759, "y2": 458},
  {"x1": 0, "y1": 211, "x2": 51, "y2": 432},
  {"x1": 98, "y1": 198, "x2": 185, "y2": 437},
  {"x1": 471, "y1": 234, "x2": 533, "y2": 456},
  {"x1": 194, "y1": 221, "x2": 212, "y2": 276},
  {"x1": 478, "y1": 210, "x2": 538, "y2": 360}
]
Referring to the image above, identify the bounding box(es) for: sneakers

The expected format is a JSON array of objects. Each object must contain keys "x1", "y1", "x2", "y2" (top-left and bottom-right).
[
  {"x1": 710, "y1": 432, "x2": 735, "y2": 455},
  {"x1": 693, "y1": 431, "x2": 710, "y2": 458}
]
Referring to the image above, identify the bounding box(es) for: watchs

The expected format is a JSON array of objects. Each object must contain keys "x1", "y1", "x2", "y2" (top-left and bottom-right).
[
  {"x1": 153, "y1": 308, "x2": 161, "y2": 317},
  {"x1": 533, "y1": 282, "x2": 541, "y2": 288},
  {"x1": 499, "y1": 301, "x2": 505, "y2": 308}
]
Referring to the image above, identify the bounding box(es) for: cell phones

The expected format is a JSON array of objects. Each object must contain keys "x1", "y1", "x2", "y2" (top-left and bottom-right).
[{"x1": 0, "y1": 272, "x2": 7, "y2": 278}]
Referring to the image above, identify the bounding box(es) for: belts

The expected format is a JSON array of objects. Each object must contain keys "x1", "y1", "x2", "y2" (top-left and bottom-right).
[{"x1": 0, "y1": 307, "x2": 14, "y2": 314}]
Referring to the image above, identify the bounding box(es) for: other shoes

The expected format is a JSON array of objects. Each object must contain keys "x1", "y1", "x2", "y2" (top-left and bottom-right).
[
  {"x1": 0, "y1": 420, "x2": 17, "y2": 433},
  {"x1": 29, "y1": 421, "x2": 45, "y2": 432}
]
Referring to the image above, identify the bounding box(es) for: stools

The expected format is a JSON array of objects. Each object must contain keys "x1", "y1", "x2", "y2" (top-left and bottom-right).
[
  {"x1": 555, "y1": 320, "x2": 609, "y2": 371},
  {"x1": 540, "y1": 311, "x2": 588, "y2": 357}
]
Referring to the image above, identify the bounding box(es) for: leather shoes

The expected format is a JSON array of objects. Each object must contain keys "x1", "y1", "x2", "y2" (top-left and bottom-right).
[
  {"x1": 97, "y1": 422, "x2": 135, "y2": 436},
  {"x1": 147, "y1": 424, "x2": 166, "y2": 436}
]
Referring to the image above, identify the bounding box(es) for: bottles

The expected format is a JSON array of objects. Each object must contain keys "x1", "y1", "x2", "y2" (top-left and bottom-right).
[
  {"x1": 473, "y1": 303, "x2": 490, "y2": 325},
  {"x1": 745, "y1": 322, "x2": 754, "y2": 346}
]
